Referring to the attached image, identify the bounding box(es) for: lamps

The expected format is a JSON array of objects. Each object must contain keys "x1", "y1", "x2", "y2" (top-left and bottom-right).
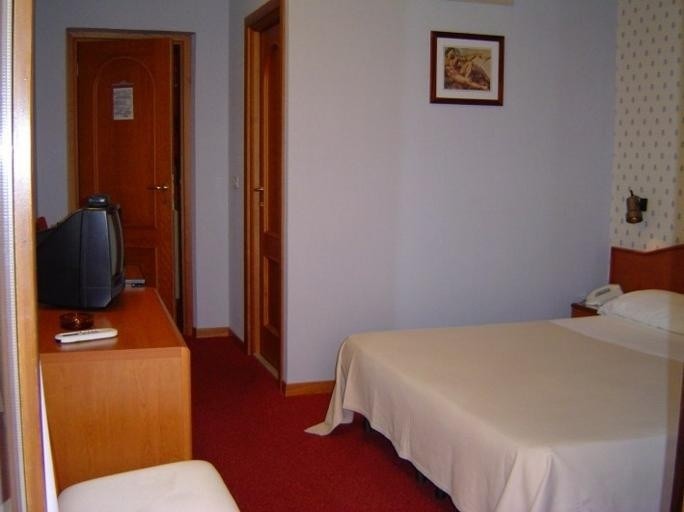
[{"x1": 625, "y1": 187, "x2": 648, "y2": 224}]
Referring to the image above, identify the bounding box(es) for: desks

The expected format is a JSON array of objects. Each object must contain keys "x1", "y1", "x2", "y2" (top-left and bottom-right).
[{"x1": 38, "y1": 288, "x2": 192, "y2": 498}]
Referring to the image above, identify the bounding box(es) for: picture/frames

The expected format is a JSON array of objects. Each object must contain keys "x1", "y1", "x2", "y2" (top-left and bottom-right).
[{"x1": 430, "y1": 32, "x2": 503, "y2": 107}]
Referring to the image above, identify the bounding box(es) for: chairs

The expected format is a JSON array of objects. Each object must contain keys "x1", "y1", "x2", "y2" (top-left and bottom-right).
[{"x1": 38, "y1": 361, "x2": 241, "y2": 512}]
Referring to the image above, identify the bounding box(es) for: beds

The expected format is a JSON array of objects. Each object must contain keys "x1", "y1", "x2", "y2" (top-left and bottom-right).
[{"x1": 304, "y1": 242, "x2": 684, "y2": 512}]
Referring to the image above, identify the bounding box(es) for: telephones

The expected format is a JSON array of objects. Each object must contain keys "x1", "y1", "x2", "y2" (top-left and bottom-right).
[{"x1": 584, "y1": 284, "x2": 623, "y2": 305}]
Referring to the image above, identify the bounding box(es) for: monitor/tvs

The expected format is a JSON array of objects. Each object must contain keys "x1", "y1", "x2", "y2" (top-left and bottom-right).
[{"x1": 36, "y1": 202, "x2": 126, "y2": 312}]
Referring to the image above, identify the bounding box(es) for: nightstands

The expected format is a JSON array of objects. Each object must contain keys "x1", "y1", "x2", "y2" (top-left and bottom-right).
[{"x1": 572, "y1": 299, "x2": 599, "y2": 317}]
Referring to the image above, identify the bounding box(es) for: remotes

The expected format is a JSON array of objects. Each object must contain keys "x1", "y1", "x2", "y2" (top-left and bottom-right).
[
  {"x1": 54, "y1": 327, "x2": 118, "y2": 344},
  {"x1": 87, "y1": 194, "x2": 108, "y2": 208}
]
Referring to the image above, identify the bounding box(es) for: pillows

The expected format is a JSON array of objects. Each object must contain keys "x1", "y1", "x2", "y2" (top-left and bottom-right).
[{"x1": 606, "y1": 286, "x2": 684, "y2": 334}]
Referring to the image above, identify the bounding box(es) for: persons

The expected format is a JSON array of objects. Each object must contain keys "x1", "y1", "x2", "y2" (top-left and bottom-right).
[{"x1": 444, "y1": 47, "x2": 489, "y2": 91}]
